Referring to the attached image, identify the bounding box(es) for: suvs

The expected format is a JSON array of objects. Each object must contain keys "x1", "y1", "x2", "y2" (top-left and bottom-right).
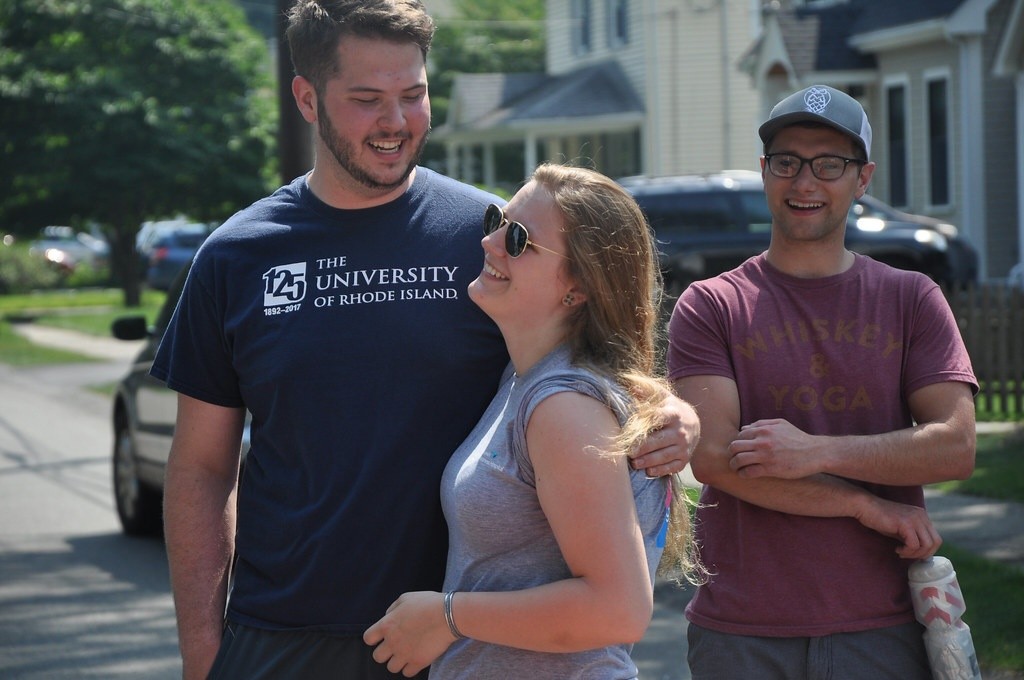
[{"x1": 612, "y1": 169, "x2": 984, "y2": 302}]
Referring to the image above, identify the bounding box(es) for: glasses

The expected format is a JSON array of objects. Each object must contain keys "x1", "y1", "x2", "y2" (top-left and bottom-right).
[
  {"x1": 483, "y1": 203, "x2": 575, "y2": 262},
  {"x1": 764, "y1": 153, "x2": 868, "y2": 180}
]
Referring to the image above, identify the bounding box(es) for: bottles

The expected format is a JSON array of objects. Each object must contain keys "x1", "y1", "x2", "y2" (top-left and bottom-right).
[{"x1": 907, "y1": 545, "x2": 983, "y2": 680}]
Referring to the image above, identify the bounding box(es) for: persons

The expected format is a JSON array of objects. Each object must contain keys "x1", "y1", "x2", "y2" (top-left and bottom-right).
[
  {"x1": 363, "y1": 163, "x2": 672, "y2": 678},
  {"x1": 143, "y1": 0, "x2": 701, "y2": 680},
  {"x1": 663, "y1": 84, "x2": 980, "y2": 678}
]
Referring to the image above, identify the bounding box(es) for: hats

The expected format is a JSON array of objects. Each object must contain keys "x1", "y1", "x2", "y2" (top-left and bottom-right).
[{"x1": 758, "y1": 84, "x2": 873, "y2": 164}]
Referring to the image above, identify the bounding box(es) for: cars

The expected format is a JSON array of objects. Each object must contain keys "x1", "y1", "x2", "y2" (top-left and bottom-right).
[
  {"x1": 107, "y1": 256, "x2": 194, "y2": 534},
  {"x1": 22, "y1": 221, "x2": 215, "y2": 288}
]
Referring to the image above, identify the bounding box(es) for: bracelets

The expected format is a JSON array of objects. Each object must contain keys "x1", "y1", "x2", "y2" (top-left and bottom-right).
[{"x1": 444, "y1": 591, "x2": 466, "y2": 641}]
